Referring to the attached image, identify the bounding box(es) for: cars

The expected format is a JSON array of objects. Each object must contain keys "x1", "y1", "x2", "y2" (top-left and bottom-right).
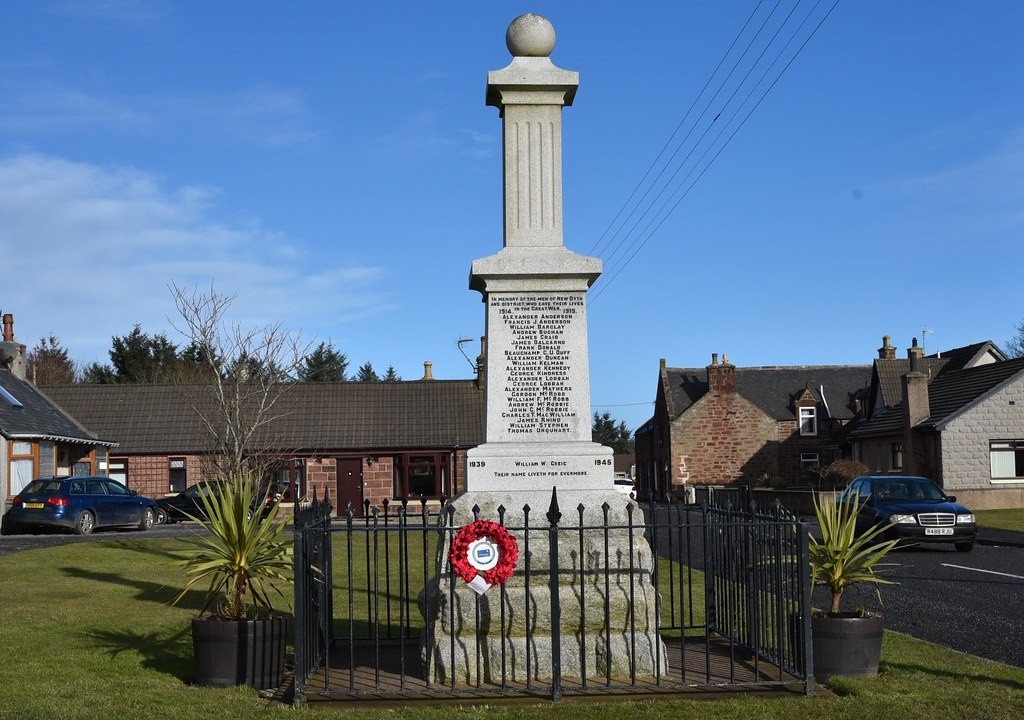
[
  {"x1": 836, "y1": 476, "x2": 979, "y2": 552},
  {"x1": 614, "y1": 478, "x2": 637, "y2": 500},
  {"x1": 156, "y1": 480, "x2": 279, "y2": 525},
  {"x1": 12, "y1": 475, "x2": 159, "y2": 534}
]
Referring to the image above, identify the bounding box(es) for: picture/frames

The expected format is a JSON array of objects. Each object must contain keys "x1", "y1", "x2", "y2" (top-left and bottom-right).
[{"x1": 792, "y1": 483, "x2": 919, "y2": 681}]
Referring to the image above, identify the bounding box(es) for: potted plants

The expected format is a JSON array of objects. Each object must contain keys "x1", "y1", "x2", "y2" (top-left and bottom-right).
[{"x1": 149, "y1": 272, "x2": 312, "y2": 690}]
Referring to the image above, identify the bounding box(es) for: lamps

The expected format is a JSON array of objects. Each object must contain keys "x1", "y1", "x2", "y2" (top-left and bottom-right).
[{"x1": 367, "y1": 457, "x2": 373, "y2": 468}]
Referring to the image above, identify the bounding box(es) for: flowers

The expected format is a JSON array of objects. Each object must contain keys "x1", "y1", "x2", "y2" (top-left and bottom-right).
[{"x1": 449, "y1": 521, "x2": 521, "y2": 590}]
